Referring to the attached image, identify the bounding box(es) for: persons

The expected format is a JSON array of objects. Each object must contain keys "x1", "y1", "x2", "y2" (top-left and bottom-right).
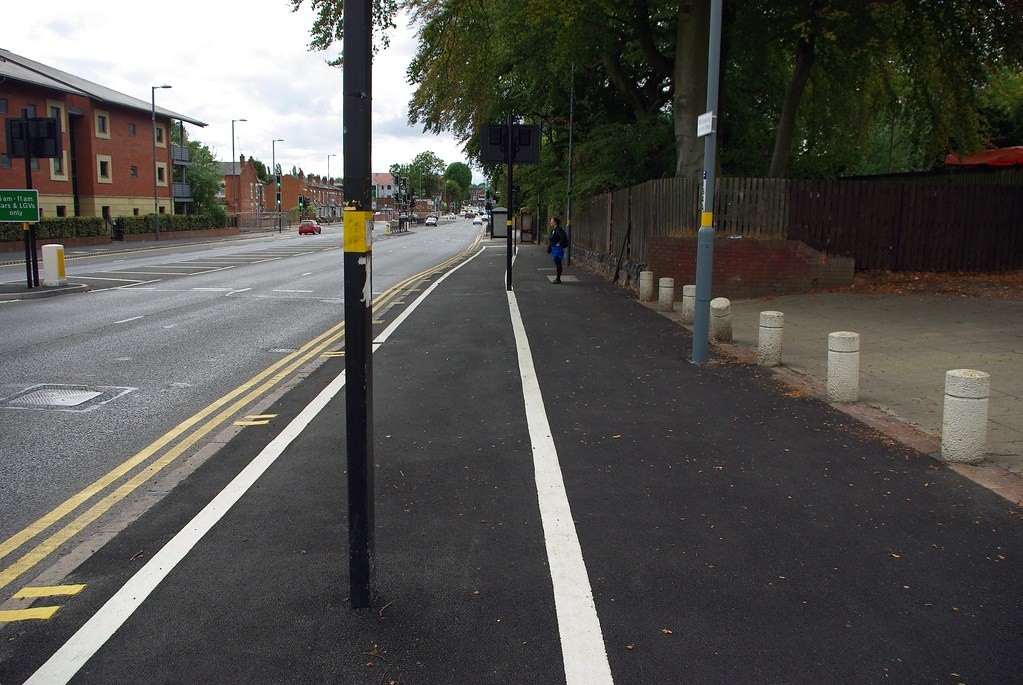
[
  {"x1": 548, "y1": 217, "x2": 568, "y2": 283},
  {"x1": 410, "y1": 213, "x2": 414, "y2": 224}
]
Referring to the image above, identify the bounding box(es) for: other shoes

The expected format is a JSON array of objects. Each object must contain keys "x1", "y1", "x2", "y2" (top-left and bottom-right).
[{"x1": 552, "y1": 279, "x2": 560, "y2": 283}]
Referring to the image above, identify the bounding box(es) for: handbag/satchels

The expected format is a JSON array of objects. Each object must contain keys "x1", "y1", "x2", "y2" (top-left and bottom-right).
[{"x1": 547, "y1": 246, "x2": 551, "y2": 253}]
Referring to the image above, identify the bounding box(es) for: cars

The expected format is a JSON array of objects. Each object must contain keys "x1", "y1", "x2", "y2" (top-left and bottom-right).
[
  {"x1": 446, "y1": 213, "x2": 456, "y2": 219},
  {"x1": 473, "y1": 217, "x2": 482, "y2": 225},
  {"x1": 482, "y1": 215, "x2": 491, "y2": 222},
  {"x1": 298, "y1": 219, "x2": 321, "y2": 234},
  {"x1": 400, "y1": 214, "x2": 419, "y2": 222},
  {"x1": 425, "y1": 214, "x2": 439, "y2": 226},
  {"x1": 460, "y1": 207, "x2": 491, "y2": 218}
]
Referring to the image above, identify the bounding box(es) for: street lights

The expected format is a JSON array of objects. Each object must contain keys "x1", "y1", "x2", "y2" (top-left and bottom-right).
[
  {"x1": 445, "y1": 180, "x2": 451, "y2": 213},
  {"x1": 232, "y1": 119, "x2": 248, "y2": 226},
  {"x1": 420, "y1": 169, "x2": 426, "y2": 222},
  {"x1": 326, "y1": 155, "x2": 337, "y2": 225},
  {"x1": 272, "y1": 138, "x2": 285, "y2": 228},
  {"x1": 151, "y1": 86, "x2": 173, "y2": 242}
]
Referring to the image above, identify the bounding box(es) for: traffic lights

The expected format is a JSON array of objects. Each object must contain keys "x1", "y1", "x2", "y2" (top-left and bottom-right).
[
  {"x1": 402, "y1": 195, "x2": 406, "y2": 205},
  {"x1": 411, "y1": 200, "x2": 416, "y2": 207},
  {"x1": 299, "y1": 195, "x2": 310, "y2": 208},
  {"x1": 394, "y1": 175, "x2": 399, "y2": 186},
  {"x1": 276, "y1": 175, "x2": 282, "y2": 205},
  {"x1": 394, "y1": 193, "x2": 399, "y2": 203},
  {"x1": 401, "y1": 177, "x2": 407, "y2": 189}
]
